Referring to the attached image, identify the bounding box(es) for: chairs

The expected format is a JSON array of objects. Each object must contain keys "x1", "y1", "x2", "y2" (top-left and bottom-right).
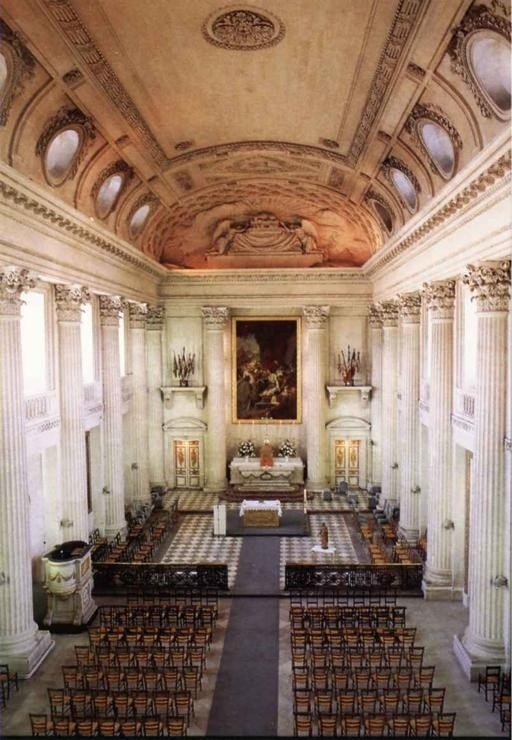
[
  {"x1": 289, "y1": 585, "x2": 510, "y2": 736},
  {"x1": 89, "y1": 496, "x2": 178, "y2": 564},
  {"x1": 322, "y1": 481, "x2": 427, "y2": 564},
  {"x1": 0, "y1": 583, "x2": 219, "y2": 735}
]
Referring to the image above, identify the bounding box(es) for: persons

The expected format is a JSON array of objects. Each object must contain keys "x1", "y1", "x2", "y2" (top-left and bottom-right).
[
  {"x1": 317, "y1": 522, "x2": 328, "y2": 549},
  {"x1": 237, "y1": 352, "x2": 296, "y2": 418}
]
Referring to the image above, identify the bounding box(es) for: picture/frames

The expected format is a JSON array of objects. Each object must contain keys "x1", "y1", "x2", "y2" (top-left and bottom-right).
[{"x1": 231, "y1": 315, "x2": 303, "y2": 424}]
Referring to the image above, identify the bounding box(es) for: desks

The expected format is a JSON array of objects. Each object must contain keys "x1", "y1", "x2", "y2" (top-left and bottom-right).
[
  {"x1": 238, "y1": 499, "x2": 282, "y2": 528},
  {"x1": 228, "y1": 456, "x2": 305, "y2": 492}
]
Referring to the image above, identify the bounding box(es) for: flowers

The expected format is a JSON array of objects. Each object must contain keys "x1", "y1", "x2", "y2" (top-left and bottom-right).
[
  {"x1": 236, "y1": 439, "x2": 256, "y2": 457},
  {"x1": 277, "y1": 438, "x2": 297, "y2": 457}
]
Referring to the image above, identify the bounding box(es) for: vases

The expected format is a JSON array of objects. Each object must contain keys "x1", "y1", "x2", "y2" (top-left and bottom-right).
[
  {"x1": 285, "y1": 455, "x2": 288, "y2": 464},
  {"x1": 244, "y1": 455, "x2": 249, "y2": 463}
]
